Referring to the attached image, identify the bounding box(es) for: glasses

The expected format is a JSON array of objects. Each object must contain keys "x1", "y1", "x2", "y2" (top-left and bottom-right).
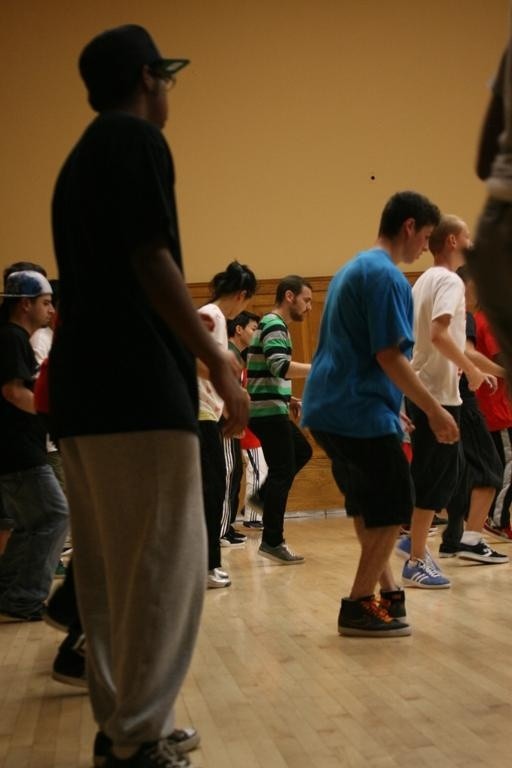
[{"x1": 158, "y1": 74, "x2": 182, "y2": 90}]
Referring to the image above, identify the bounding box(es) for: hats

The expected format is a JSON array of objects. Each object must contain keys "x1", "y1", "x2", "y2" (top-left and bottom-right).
[
  {"x1": 79, "y1": 21, "x2": 194, "y2": 108},
  {"x1": 0, "y1": 271, "x2": 55, "y2": 300}
]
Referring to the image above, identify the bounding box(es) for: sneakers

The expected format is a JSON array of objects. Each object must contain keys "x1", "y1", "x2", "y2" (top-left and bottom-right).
[
  {"x1": 207, "y1": 575, "x2": 232, "y2": 589},
  {"x1": 218, "y1": 519, "x2": 264, "y2": 546},
  {"x1": 0, "y1": 602, "x2": 49, "y2": 623},
  {"x1": 92, "y1": 726, "x2": 201, "y2": 765},
  {"x1": 257, "y1": 539, "x2": 305, "y2": 565},
  {"x1": 52, "y1": 639, "x2": 90, "y2": 688},
  {"x1": 101, "y1": 739, "x2": 193, "y2": 768},
  {"x1": 214, "y1": 567, "x2": 228, "y2": 578},
  {"x1": 338, "y1": 509, "x2": 510, "y2": 639}
]
